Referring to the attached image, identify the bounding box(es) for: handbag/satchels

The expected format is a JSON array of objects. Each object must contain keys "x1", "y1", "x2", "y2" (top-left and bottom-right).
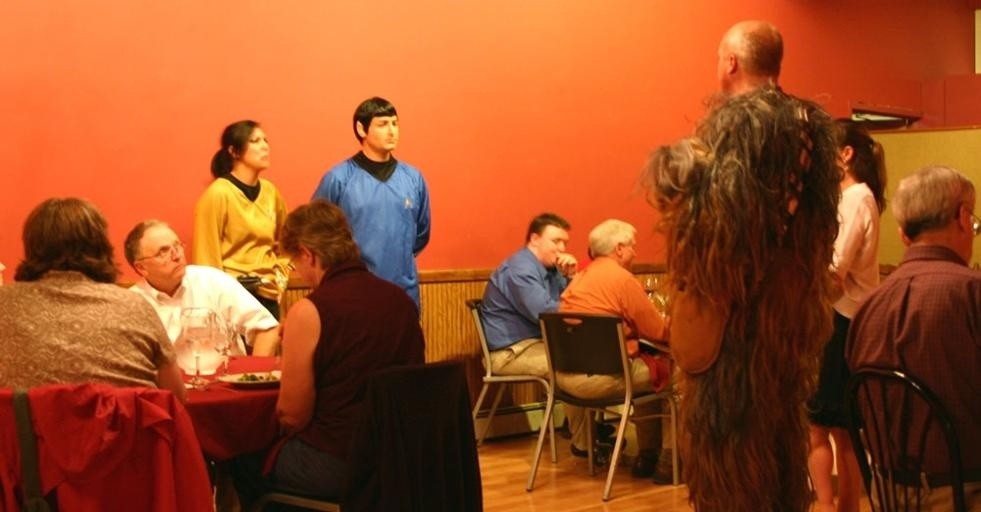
[{"x1": 667, "y1": 277, "x2": 734, "y2": 375}]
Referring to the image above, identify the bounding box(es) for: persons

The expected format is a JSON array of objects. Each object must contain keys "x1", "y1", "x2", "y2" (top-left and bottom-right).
[
  {"x1": 125, "y1": 218, "x2": 281, "y2": 377},
  {"x1": 843, "y1": 164, "x2": 981, "y2": 477},
  {"x1": 479, "y1": 211, "x2": 627, "y2": 459},
  {"x1": 553, "y1": 218, "x2": 686, "y2": 485},
  {"x1": 190, "y1": 119, "x2": 286, "y2": 322},
  {"x1": 0, "y1": 196, "x2": 188, "y2": 406},
  {"x1": 308, "y1": 97, "x2": 430, "y2": 320},
  {"x1": 803, "y1": 115, "x2": 887, "y2": 512},
  {"x1": 641, "y1": 21, "x2": 838, "y2": 511},
  {"x1": 263, "y1": 199, "x2": 426, "y2": 504}
]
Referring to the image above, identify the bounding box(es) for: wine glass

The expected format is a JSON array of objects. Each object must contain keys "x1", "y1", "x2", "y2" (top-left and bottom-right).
[
  {"x1": 644, "y1": 274, "x2": 658, "y2": 296},
  {"x1": 215, "y1": 322, "x2": 236, "y2": 374},
  {"x1": 181, "y1": 308, "x2": 211, "y2": 387}
]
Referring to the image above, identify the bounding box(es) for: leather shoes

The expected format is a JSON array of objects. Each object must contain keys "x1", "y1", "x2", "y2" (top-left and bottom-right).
[
  {"x1": 562, "y1": 417, "x2": 615, "y2": 439},
  {"x1": 570, "y1": 437, "x2": 626, "y2": 457},
  {"x1": 633, "y1": 457, "x2": 655, "y2": 478},
  {"x1": 652, "y1": 459, "x2": 683, "y2": 484}
]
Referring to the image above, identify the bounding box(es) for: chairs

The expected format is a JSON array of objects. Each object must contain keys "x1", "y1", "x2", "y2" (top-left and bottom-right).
[
  {"x1": 272, "y1": 359, "x2": 469, "y2": 512},
  {"x1": 0, "y1": 386, "x2": 174, "y2": 512},
  {"x1": 464, "y1": 297, "x2": 558, "y2": 463},
  {"x1": 842, "y1": 366, "x2": 965, "y2": 512},
  {"x1": 526, "y1": 312, "x2": 681, "y2": 502}
]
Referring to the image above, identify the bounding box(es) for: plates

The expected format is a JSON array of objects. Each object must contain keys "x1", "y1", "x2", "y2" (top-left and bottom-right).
[{"x1": 218, "y1": 372, "x2": 281, "y2": 385}]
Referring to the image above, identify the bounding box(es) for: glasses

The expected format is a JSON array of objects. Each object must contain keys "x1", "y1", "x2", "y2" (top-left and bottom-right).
[
  {"x1": 964, "y1": 205, "x2": 981, "y2": 236},
  {"x1": 136, "y1": 241, "x2": 187, "y2": 262},
  {"x1": 286, "y1": 247, "x2": 302, "y2": 271}
]
